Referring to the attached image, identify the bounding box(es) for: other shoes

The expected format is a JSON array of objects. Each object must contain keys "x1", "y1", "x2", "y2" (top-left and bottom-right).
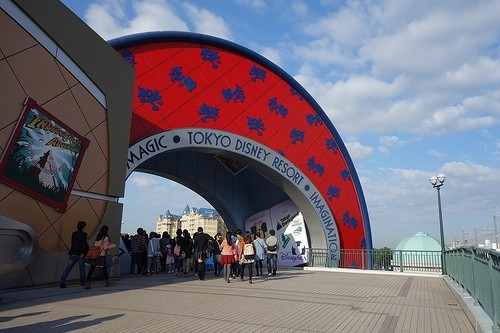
[
  {"x1": 129, "y1": 274, "x2": 135, "y2": 277},
  {"x1": 154, "y1": 269, "x2": 205, "y2": 281},
  {"x1": 59, "y1": 282, "x2": 67, "y2": 288},
  {"x1": 85, "y1": 281, "x2": 92, "y2": 289},
  {"x1": 248, "y1": 280, "x2": 252, "y2": 284},
  {"x1": 147, "y1": 272, "x2": 150, "y2": 276},
  {"x1": 137, "y1": 274, "x2": 142, "y2": 277},
  {"x1": 239, "y1": 278, "x2": 243, "y2": 281},
  {"x1": 80, "y1": 282, "x2": 85, "y2": 286},
  {"x1": 268, "y1": 272, "x2": 276, "y2": 276},
  {"x1": 256, "y1": 270, "x2": 263, "y2": 276},
  {"x1": 104, "y1": 280, "x2": 112, "y2": 287}
]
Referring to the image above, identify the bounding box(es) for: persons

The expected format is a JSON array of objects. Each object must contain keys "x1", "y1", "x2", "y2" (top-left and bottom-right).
[
  {"x1": 130, "y1": 228, "x2": 146, "y2": 278},
  {"x1": 239, "y1": 233, "x2": 256, "y2": 284},
  {"x1": 84, "y1": 225, "x2": 112, "y2": 289},
  {"x1": 172, "y1": 229, "x2": 193, "y2": 278},
  {"x1": 193, "y1": 227, "x2": 212, "y2": 280},
  {"x1": 59, "y1": 221, "x2": 89, "y2": 288},
  {"x1": 143, "y1": 230, "x2": 174, "y2": 276},
  {"x1": 253, "y1": 232, "x2": 267, "y2": 276},
  {"x1": 213, "y1": 233, "x2": 224, "y2": 277},
  {"x1": 219, "y1": 231, "x2": 234, "y2": 283},
  {"x1": 266, "y1": 230, "x2": 278, "y2": 277},
  {"x1": 230, "y1": 229, "x2": 244, "y2": 278},
  {"x1": 122, "y1": 234, "x2": 134, "y2": 252}
]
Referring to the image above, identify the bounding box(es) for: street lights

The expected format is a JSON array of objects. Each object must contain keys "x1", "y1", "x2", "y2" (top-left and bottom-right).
[{"x1": 428, "y1": 173, "x2": 447, "y2": 275}]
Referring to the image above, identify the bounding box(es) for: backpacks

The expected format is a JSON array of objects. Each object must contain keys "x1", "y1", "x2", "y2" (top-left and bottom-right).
[{"x1": 243, "y1": 239, "x2": 255, "y2": 259}]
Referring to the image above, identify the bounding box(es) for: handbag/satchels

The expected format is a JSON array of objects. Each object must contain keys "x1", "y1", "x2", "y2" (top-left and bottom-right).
[
  {"x1": 174, "y1": 244, "x2": 181, "y2": 256},
  {"x1": 153, "y1": 250, "x2": 160, "y2": 258},
  {"x1": 87, "y1": 245, "x2": 102, "y2": 260},
  {"x1": 268, "y1": 244, "x2": 277, "y2": 251},
  {"x1": 181, "y1": 251, "x2": 186, "y2": 259}
]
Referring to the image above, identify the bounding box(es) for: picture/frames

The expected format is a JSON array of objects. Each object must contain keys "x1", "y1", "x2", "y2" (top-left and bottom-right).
[{"x1": 0, "y1": 97, "x2": 91, "y2": 213}]
